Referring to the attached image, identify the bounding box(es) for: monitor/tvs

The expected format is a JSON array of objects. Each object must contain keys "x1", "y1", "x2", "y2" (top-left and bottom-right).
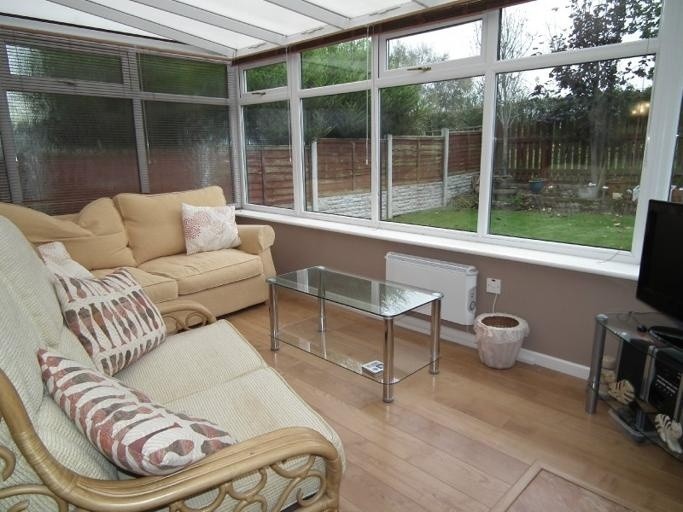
[{"x1": 635, "y1": 199, "x2": 683, "y2": 351}]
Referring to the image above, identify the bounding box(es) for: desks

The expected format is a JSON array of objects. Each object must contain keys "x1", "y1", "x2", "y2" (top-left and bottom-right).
[{"x1": 584, "y1": 311, "x2": 682, "y2": 462}]
